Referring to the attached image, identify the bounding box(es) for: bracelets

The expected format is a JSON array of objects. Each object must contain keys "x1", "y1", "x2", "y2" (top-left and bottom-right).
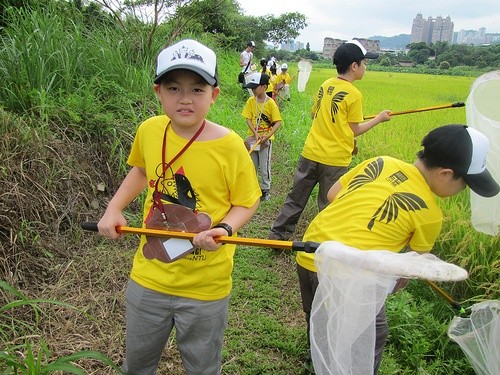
[{"x1": 212, "y1": 223, "x2": 232, "y2": 245}]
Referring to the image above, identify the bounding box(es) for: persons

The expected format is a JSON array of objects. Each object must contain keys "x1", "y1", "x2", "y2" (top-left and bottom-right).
[
  {"x1": 240, "y1": 41, "x2": 291, "y2": 101},
  {"x1": 268, "y1": 40, "x2": 392, "y2": 252},
  {"x1": 296, "y1": 124, "x2": 500, "y2": 375},
  {"x1": 241, "y1": 73, "x2": 283, "y2": 199},
  {"x1": 97, "y1": 40, "x2": 263, "y2": 375}
]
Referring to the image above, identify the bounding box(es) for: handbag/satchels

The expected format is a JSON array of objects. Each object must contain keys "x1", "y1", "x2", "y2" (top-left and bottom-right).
[{"x1": 238, "y1": 71, "x2": 245, "y2": 83}]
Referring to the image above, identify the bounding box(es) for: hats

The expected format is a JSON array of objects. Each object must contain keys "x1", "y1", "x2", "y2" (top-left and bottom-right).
[
  {"x1": 280, "y1": 62, "x2": 288, "y2": 70},
  {"x1": 333, "y1": 39, "x2": 380, "y2": 67},
  {"x1": 154, "y1": 38, "x2": 219, "y2": 87},
  {"x1": 243, "y1": 72, "x2": 269, "y2": 88},
  {"x1": 422, "y1": 122, "x2": 500, "y2": 198},
  {"x1": 247, "y1": 40, "x2": 259, "y2": 49}
]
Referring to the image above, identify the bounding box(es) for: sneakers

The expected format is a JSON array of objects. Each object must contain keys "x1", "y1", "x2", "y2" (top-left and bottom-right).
[{"x1": 260, "y1": 189, "x2": 270, "y2": 202}]
